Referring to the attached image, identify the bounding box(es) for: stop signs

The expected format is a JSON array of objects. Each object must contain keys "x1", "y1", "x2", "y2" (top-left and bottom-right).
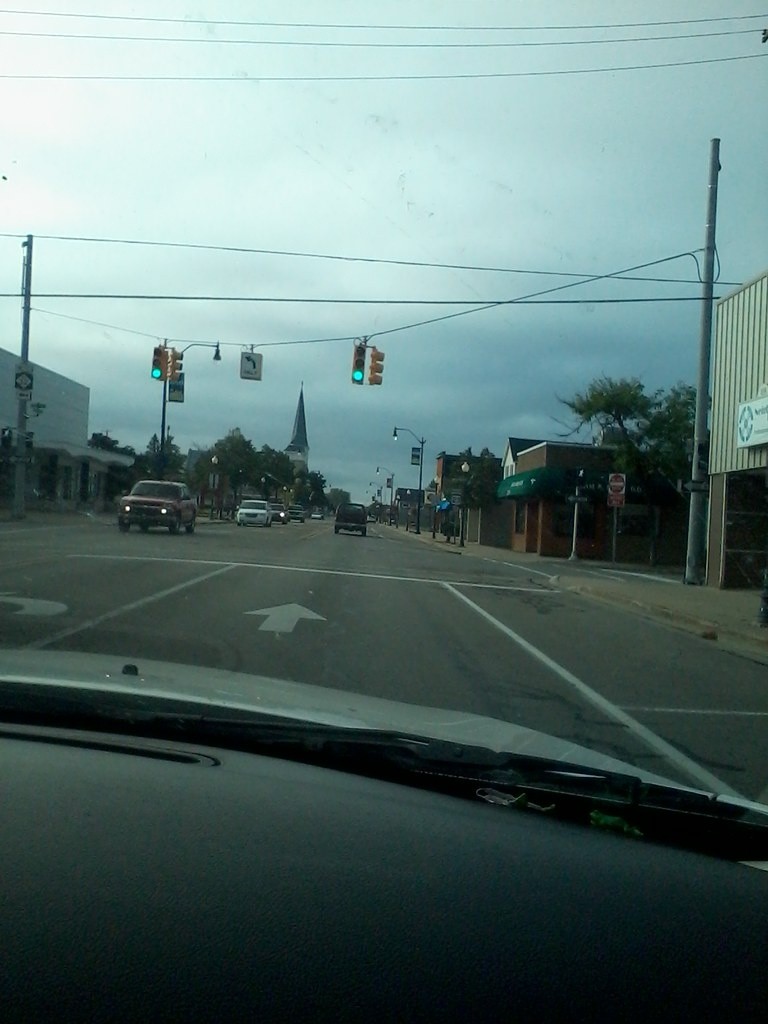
[{"x1": 608, "y1": 474, "x2": 626, "y2": 494}]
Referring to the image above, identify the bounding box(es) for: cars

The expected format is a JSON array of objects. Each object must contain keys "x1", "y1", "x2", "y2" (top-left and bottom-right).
[
  {"x1": 268, "y1": 503, "x2": 290, "y2": 525},
  {"x1": 311, "y1": 512, "x2": 324, "y2": 519}
]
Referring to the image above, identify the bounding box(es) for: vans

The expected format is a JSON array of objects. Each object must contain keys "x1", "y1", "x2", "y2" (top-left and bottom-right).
[
  {"x1": 335, "y1": 503, "x2": 367, "y2": 536},
  {"x1": 237, "y1": 499, "x2": 273, "y2": 526}
]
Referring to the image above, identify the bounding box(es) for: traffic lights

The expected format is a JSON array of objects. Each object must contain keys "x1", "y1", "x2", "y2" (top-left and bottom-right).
[
  {"x1": 369, "y1": 349, "x2": 384, "y2": 385},
  {"x1": 151, "y1": 347, "x2": 169, "y2": 381},
  {"x1": 352, "y1": 345, "x2": 366, "y2": 385},
  {"x1": 170, "y1": 351, "x2": 183, "y2": 381}
]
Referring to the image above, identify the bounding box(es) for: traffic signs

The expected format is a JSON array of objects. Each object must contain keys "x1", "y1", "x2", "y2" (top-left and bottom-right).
[{"x1": 567, "y1": 496, "x2": 588, "y2": 503}]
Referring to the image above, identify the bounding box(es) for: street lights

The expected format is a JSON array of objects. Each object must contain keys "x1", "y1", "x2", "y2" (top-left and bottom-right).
[
  {"x1": 393, "y1": 427, "x2": 426, "y2": 533},
  {"x1": 460, "y1": 462, "x2": 470, "y2": 547},
  {"x1": 209, "y1": 455, "x2": 218, "y2": 520},
  {"x1": 406, "y1": 489, "x2": 411, "y2": 531},
  {"x1": 432, "y1": 474, "x2": 441, "y2": 538},
  {"x1": 376, "y1": 467, "x2": 394, "y2": 526},
  {"x1": 369, "y1": 482, "x2": 384, "y2": 523}
]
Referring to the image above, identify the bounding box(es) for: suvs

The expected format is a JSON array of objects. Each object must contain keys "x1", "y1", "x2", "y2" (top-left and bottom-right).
[
  {"x1": 287, "y1": 504, "x2": 305, "y2": 523},
  {"x1": 118, "y1": 480, "x2": 198, "y2": 533}
]
